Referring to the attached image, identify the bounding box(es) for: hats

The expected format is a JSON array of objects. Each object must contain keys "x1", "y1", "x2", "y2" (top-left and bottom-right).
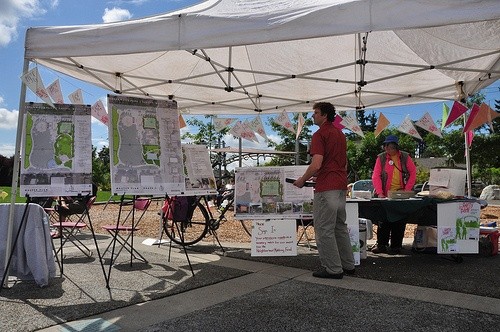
[{"x1": 383, "y1": 135, "x2": 399, "y2": 146}]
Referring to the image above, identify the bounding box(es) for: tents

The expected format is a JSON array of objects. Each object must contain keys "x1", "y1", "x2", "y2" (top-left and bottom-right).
[{"x1": 1, "y1": 0, "x2": 500, "y2": 292}]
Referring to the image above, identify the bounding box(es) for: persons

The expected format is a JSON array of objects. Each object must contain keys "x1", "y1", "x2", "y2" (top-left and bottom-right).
[
  {"x1": 293, "y1": 101, "x2": 356, "y2": 279},
  {"x1": 51, "y1": 185, "x2": 98, "y2": 239},
  {"x1": 371, "y1": 134, "x2": 416, "y2": 255}
]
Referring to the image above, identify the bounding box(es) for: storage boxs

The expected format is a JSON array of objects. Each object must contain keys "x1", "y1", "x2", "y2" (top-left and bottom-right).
[{"x1": 479, "y1": 227, "x2": 499, "y2": 256}]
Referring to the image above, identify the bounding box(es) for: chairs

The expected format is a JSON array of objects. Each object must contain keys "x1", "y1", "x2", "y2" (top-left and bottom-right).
[
  {"x1": 351, "y1": 180, "x2": 376, "y2": 198},
  {"x1": 101, "y1": 196, "x2": 152, "y2": 267},
  {"x1": 44, "y1": 205, "x2": 69, "y2": 234},
  {"x1": 52, "y1": 196, "x2": 97, "y2": 258}
]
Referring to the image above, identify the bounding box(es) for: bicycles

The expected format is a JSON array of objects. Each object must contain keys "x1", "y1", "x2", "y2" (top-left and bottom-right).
[{"x1": 163, "y1": 189, "x2": 253, "y2": 245}]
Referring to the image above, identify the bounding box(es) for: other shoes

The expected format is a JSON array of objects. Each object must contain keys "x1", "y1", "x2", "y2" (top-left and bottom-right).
[
  {"x1": 312, "y1": 268, "x2": 355, "y2": 279},
  {"x1": 371, "y1": 243, "x2": 401, "y2": 255}
]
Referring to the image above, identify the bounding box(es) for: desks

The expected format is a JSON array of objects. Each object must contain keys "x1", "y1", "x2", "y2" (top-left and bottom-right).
[
  {"x1": 345, "y1": 195, "x2": 488, "y2": 263},
  {"x1": 0, "y1": 203, "x2": 57, "y2": 286}
]
